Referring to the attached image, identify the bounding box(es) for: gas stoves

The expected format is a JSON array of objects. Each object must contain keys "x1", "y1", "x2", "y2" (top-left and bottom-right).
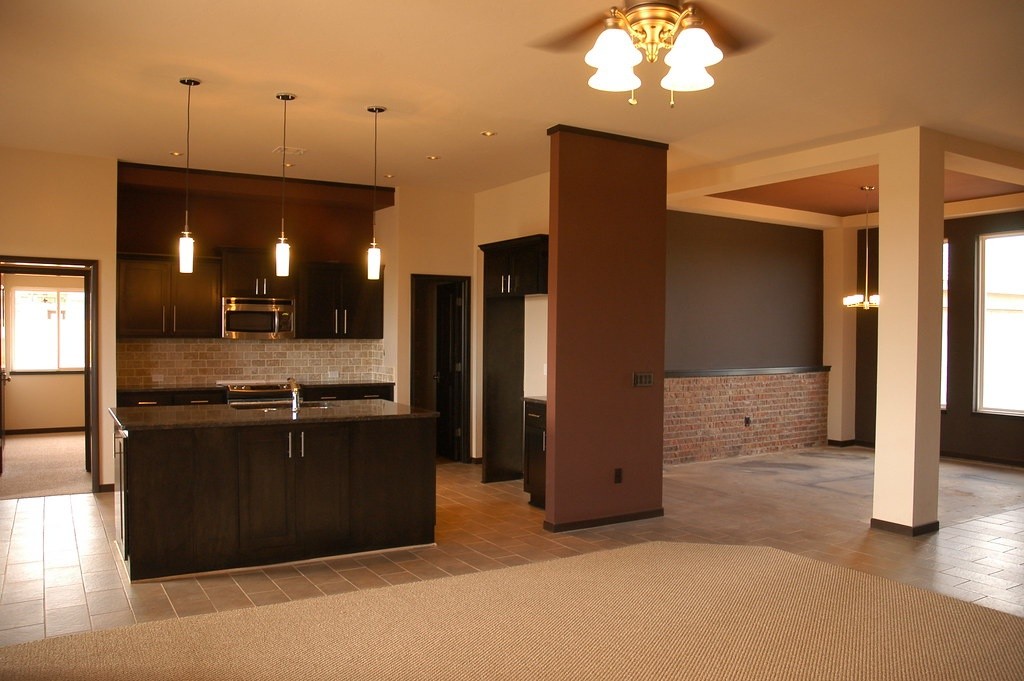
[{"x1": 225, "y1": 381, "x2": 303, "y2": 409}]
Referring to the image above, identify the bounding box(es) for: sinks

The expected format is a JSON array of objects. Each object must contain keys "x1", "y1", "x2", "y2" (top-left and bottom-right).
[{"x1": 228, "y1": 397, "x2": 334, "y2": 413}]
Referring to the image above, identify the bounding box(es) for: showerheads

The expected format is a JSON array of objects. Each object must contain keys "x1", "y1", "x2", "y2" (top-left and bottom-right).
[{"x1": 286, "y1": 376, "x2": 303, "y2": 412}]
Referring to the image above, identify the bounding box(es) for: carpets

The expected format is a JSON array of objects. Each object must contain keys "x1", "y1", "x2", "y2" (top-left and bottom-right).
[{"x1": 0, "y1": 541, "x2": 1024, "y2": 681}]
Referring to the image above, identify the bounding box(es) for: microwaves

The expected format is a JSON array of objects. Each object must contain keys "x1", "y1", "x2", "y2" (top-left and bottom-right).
[{"x1": 220, "y1": 295, "x2": 296, "y2": 341}]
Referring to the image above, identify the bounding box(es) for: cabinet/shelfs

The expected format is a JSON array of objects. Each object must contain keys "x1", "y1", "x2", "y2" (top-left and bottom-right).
[
  {"x1": 217, "y1": 251, "x2": 298, "y2": 299},
  {"x1": 293, "y1": 268, "x2": 385, "y2": 340},
  {"x1": 116, "y1": 256, "x2": 221, "y2": 338},
  {"x1": 124, "y1": 416, "x2": 439, "y2": 584},
  {"x1": 520, "y1": 401, "x2": 545, "y2": 511}
]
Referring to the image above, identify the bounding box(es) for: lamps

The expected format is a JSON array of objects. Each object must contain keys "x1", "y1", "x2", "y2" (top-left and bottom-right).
[
  {"x1": 275, "y1": 92, "x2": 297, "y2": 278},
  {"x1": 842, "y1": 186, "x2": 879, "y2": 309},
  {"x1": 367, "y1": 106, "x2": 387, "y2": 280},
  {"x1": 584, "y1": 0, "x2": 723, "y2": 107},
  {"x1": 178, "y1": 77, "x2": 200, "y2": 275}
]
